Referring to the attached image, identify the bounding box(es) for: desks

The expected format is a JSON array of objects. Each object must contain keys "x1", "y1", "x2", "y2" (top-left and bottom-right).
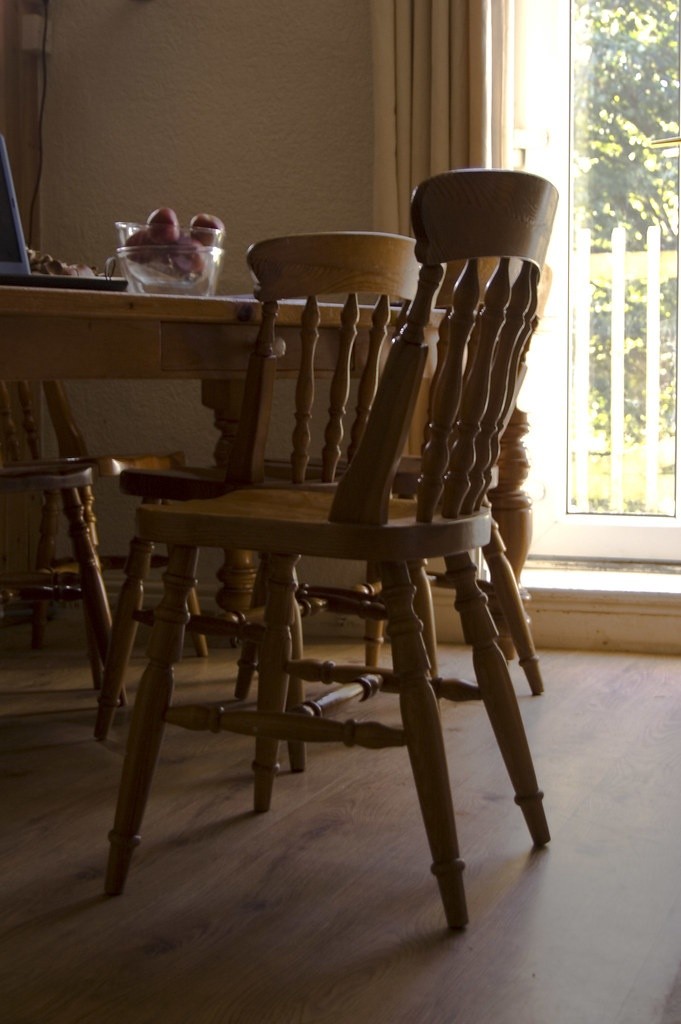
[{"x1": 0, "y1": 283, "x2": 532, "y2": 667}]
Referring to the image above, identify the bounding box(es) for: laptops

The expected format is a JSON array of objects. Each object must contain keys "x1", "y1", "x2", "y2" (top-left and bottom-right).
[{"x1": 0, "y1": 134, "x2": 129, "y2": 291}]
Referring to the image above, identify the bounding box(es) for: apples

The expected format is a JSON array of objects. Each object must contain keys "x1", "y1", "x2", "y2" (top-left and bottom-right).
[
  {"x1": 191, "y1": 214, "x2": 224, "y2": 245},
  {"x1": 147, "y1": 208, "x2": 179, "y2": 243},
  {"x1": 171, "y1": 239, "x2": 208, "y2": 274},
  {"x1": 125, "y1": 230, "x2": 157, "y2": 263}
]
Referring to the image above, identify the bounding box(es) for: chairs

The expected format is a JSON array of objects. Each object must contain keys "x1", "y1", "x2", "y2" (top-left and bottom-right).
[{"x1": 0, "y1": 170, "x2": 561, "y2": 930}]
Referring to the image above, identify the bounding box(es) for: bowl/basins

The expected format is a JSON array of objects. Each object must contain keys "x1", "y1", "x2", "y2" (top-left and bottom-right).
[
  {"x1": 117, "y1": 245, "x2": 225, "y2": 297},
  {"x1": 117, "y1": 221, "x2": 224, "y2": 287}
]
